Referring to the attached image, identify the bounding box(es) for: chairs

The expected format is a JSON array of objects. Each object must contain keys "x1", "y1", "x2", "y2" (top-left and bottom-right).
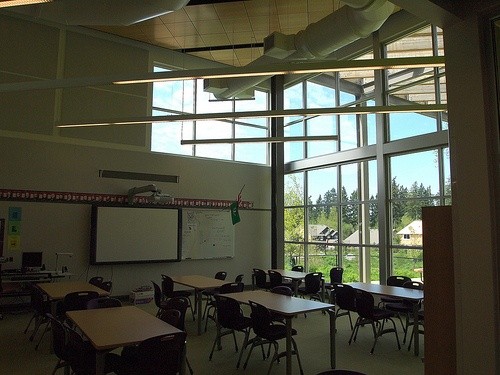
[{"x1": 25, "y1": 264, "x2": 424, "y2": 375}]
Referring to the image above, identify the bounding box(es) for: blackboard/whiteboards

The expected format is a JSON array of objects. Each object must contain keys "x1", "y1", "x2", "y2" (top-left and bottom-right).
[
  {"x1": 181, "y1": 207, "x2": 236, "y2": 261},
  {"x1": 185, "y1": 258, "x2": 191, "y2": 259}
]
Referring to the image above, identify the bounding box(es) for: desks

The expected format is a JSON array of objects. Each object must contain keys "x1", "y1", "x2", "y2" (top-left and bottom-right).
[
  {"x1": 66, "y1": 306, "x2": 184, "y2": 375},
  {"x1": 331, "y1": 282, "x2": 424, "y2": 356},
  {"x1": 251, "y1": 269, "x2": 325, "y2": 317},
  {"x1": 218, "y1": 290, "x2": 335, "y2": 375},
  {"x1": 37, "y1": 279, "x2": 110, "y2": 352},
  {"x1": 171, "y1": 275, "x2": 231, "y2": 335},
  {"x1": 0, "y1": 270, "x2": 72, "y2": 282}
]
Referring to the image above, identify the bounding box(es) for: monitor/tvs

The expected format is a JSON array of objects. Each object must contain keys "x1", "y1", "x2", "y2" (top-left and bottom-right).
[{"x1": 21, "y1": 251, "x2": 43, "y2": 269}]
